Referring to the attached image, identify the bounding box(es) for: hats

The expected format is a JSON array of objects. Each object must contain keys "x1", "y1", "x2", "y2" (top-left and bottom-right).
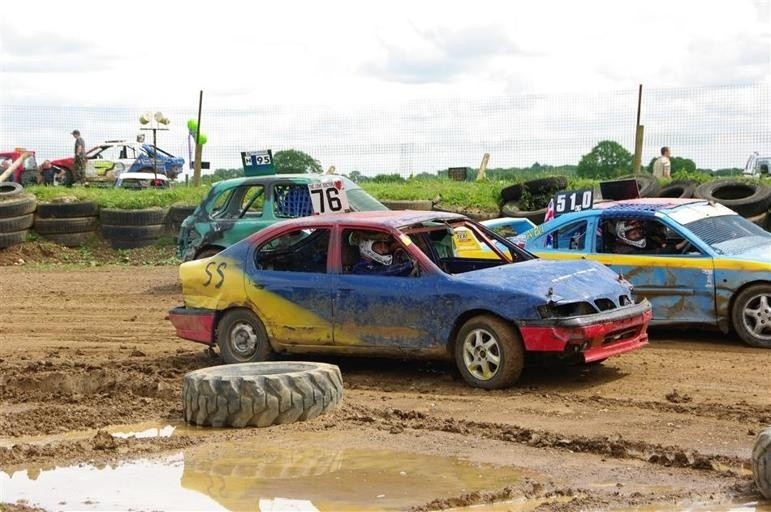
[{"x1": 71, "y1": 131, "x2": 80, "y2": 135}]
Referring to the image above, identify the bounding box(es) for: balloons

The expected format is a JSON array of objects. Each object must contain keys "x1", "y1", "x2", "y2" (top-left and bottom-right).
[
  {"x1": 196, "y1": 135, "x2": 207, "y2": 145},
  {"x1": 188, "y1": 119, "x2": 198, "y2": 131}
]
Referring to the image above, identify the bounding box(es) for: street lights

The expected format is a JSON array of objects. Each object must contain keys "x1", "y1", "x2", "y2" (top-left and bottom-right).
[{"x1": 135, "y1": 109, "x2": 171, "y2": 189}]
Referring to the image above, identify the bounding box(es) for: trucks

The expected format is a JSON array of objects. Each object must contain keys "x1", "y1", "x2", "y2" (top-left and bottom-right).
[{"x1": 742, "y1": 151, "x2": 771, "y2": 178}]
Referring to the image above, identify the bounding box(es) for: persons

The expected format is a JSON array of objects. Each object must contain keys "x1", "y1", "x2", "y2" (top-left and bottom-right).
[
  {"x1": 37, "y1": 159, "x2": 66, "y2": 187},
  {"x1": 652, "y1": 146, "x2": 671, "y2": 179},
  {"x1": 71, "y1": 130, "x2": 87, "y2": 186},
  {"x1": 350, "y1": 230, "x2": 419, "y2": 277},
  {"x1": 575, "y1": 218, "x2": 612, "y2": 253},
  {"x1": 646, "y1": 218, "x2": 687, "y2": 250},
  {"x1": 611, "y1": 218, "x2": 662, "y2": 253},
  {"x1": 0, "y1": 160, "x2": 17, "y2": 182},
  {"x1": 297, "y1": 232, "x2": 349, "y2": 272}
]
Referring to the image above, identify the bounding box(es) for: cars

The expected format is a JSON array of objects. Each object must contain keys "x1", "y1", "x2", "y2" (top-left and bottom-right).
[
  {"x1": 0, "y1": 137, "x2": 182, "y2": 187},
  {"x1": 171, "y1": 171, "x2": 395, "y2": 264},
  {"x1": 476, "y1": 194, "x2": 771, "y2": 349},
  {"x1": 168, "y1": 209, "x2": 652, "y2": 386},
  {"x1": 176, "y1": 438, "x2": 524, "y2": 511}
]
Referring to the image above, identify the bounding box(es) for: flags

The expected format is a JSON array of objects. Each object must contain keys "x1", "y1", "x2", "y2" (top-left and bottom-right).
[{"x1": 543, "y1": 198, "x2": 554, "y2": 246}]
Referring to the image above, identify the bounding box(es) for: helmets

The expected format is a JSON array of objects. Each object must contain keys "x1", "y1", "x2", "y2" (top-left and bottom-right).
[
  {"x1": 359, "y1": 233, "x2": 393, "y2": 266},
  {"x1": 349, "y1": 231, "x2": 359, "y2": 246},
  {"x1": 615, "y1": 220, "x2": 646, "y2": 248}
]
